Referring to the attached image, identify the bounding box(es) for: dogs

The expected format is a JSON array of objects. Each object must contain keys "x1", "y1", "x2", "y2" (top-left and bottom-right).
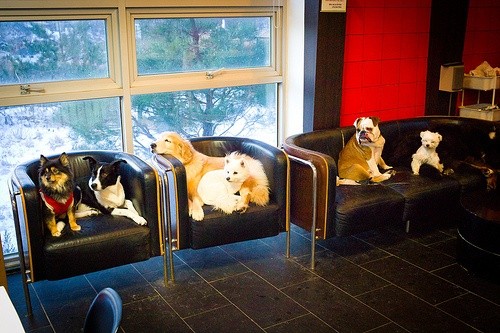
[
  {"x1": 150, "y1": 131, "x2": 270, "y2": 221},
  {"x1": 411, "y1": 130, "x2": 444, "y2": 175},
  {"x1": 37, "y1": 152, "x2": 82, "y2": 237},
  {"x1": 197, "y1": 157, "x2": 248, "y2": 214},
  {"x1": 74, "y1": 156, "x2": 147, "y2": 226},
  {"x1": 335, "y1": 115, "x2": 396, "y2": 186}
]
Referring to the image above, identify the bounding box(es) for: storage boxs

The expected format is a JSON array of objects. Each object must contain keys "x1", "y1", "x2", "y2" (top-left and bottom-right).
[
  {"x1": 458, "y1": 105, "x2": 500, "y2": 122},
  {"x1": 463, "y1": 76, "x2": 500, "y2": 91},
  {"x1": 439, "y1": 64, "x2": 464, "y2": 90}
]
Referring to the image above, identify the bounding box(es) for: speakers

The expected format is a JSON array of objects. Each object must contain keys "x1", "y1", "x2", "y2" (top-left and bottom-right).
[{"x1": 439, "y1": 65, "x2": 464, "y2": 92}]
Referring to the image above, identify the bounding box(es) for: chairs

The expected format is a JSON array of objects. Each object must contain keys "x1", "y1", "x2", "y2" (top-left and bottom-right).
[
  {"x1": 151, "y1": 137, "x2": 291, "y2": 281},
  {"x1": 7, "y1": 150, "x2": 169, "y2": 327},
  {"x1": 82, "y1": 287, "x2": 123, "y2": 333}
]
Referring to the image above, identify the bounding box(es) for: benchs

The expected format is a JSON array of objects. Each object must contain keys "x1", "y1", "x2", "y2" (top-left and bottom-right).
[{"x1": 282, "y1": 116, "x2": 500, "y2": 270}]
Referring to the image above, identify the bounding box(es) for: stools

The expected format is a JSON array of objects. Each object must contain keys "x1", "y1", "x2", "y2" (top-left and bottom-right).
[{"x1": 457, "y1": 190, "x2": 500, "y2": 272}]
